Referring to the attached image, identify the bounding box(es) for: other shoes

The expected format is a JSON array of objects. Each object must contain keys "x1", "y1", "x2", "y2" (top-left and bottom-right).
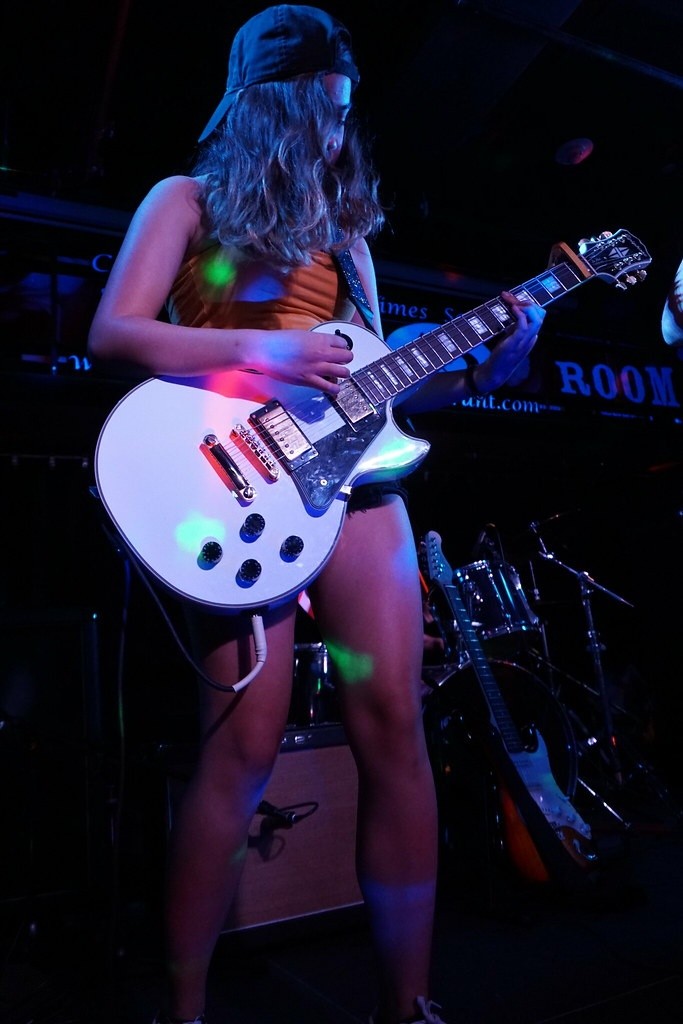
[
  {"x1": 368, "y1": 996, "x2": 446, "y2": 1024},
  {"x1": 152, "y1": 1015, "x2": 207, "y2": 1024}
]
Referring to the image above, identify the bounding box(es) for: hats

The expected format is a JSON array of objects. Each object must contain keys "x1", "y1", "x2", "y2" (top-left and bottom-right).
[{"x1": 198, "y1": 4, "x2": 359, "y2": 142}]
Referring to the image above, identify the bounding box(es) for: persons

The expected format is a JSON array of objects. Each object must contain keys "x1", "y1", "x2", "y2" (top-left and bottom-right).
[{"x1": 89, "y1": 4, "x2": 546, "y2": 1024}]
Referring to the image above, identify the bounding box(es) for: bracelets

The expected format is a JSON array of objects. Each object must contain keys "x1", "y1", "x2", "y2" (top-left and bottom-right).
[{"x1": 461, "y1": 353, "x2": 488, "y2": 401}]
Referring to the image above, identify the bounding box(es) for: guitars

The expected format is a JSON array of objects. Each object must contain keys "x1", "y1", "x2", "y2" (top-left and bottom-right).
[
  {"x1": 92, "y1": 228, "x2": 653, "y2": 622},
  {"x1": 424, "y1": 530, "x2": 593, "y2": 869}
]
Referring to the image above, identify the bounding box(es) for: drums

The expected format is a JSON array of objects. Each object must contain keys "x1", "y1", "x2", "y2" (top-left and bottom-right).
[
  {"x1": 451, "y1": 558, "x2": 541, "y2": 652},
  {"x1": 419, "y1": 657, "x2": 579, "y2": 807}
]
